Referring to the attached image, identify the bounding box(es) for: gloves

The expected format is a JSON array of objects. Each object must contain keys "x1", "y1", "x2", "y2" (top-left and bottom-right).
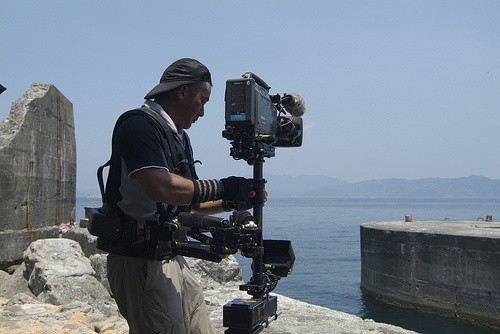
[{"x1": 220, "y1": 176, "x2": 268, "y2": 213}]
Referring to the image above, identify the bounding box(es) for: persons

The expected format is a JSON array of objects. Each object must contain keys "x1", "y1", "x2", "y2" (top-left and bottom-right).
[{"x1": 79, "y1": 58, "x2": 268, "y2": 334}]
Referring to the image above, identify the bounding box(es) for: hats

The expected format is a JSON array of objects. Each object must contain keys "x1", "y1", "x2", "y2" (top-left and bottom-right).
[{"x1": 144, "y1": 58, "x2": 211, "y2": 100}]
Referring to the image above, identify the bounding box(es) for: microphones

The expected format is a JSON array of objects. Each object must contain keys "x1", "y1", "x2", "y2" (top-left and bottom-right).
[{"x1": 283, "y1": 93, "x2": 306, "y2": 117}]
